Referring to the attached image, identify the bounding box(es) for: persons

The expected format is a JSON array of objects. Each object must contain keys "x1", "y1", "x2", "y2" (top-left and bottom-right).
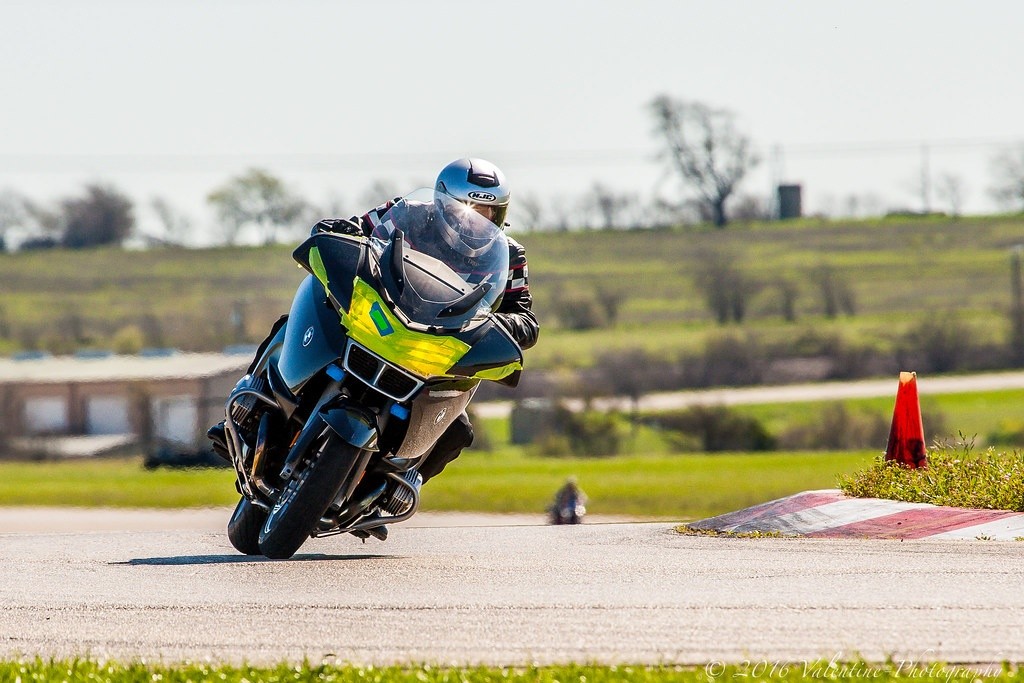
[
  {"x1": 551, "y1": 478, "x2": 586, "y2": 525},
  {"x1": 206, "y1": 158, "x2": 540, "y2": 539}
]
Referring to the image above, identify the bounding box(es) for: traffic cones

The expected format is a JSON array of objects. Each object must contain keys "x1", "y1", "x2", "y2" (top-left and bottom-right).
[{"x1": 884, "y1": 370, "x2": 928, "y2": 471}]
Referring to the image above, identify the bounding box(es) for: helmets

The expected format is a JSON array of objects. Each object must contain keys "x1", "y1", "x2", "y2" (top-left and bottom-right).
[{"x1": 434, "y1": 157, "x2": 509, "y2": 257}]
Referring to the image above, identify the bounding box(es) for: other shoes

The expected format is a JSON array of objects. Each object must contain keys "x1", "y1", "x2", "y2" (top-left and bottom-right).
[
  {"x1": 360, "y1": 509, "x2": 388, "y2": 541},
  {"x1": 206, "y1": 421, "x2": 243, "y2": 454}
]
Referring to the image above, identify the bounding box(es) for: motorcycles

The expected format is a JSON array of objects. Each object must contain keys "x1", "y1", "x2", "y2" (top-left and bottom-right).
[{"x1": 212, "y1": 189, "x2": 524, "y2": 561}]
